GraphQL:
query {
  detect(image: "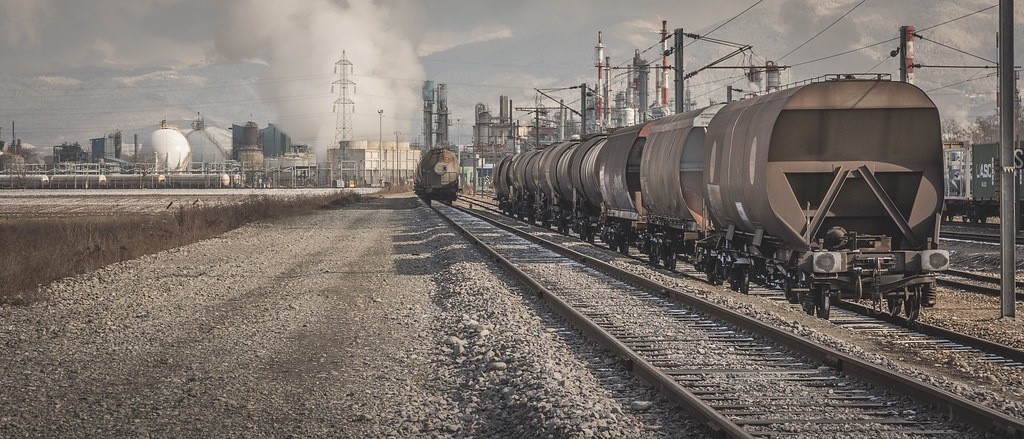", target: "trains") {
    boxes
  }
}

[414,147,462,205]
[492,72,948,321]
